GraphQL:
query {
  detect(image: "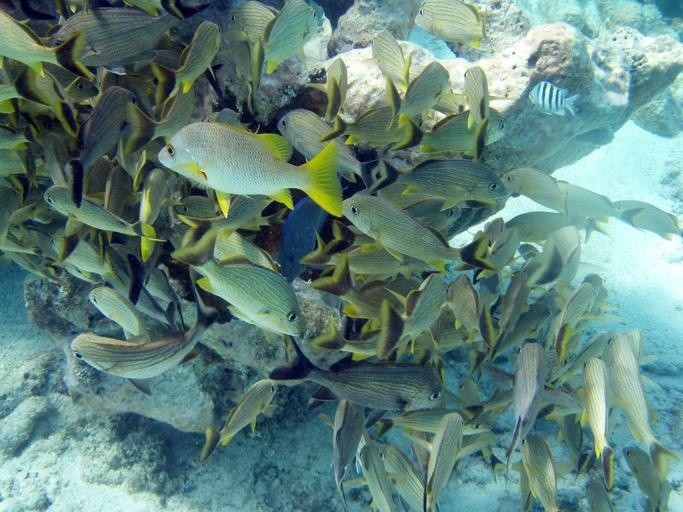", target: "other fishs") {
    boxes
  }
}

[0,0,682,512]
